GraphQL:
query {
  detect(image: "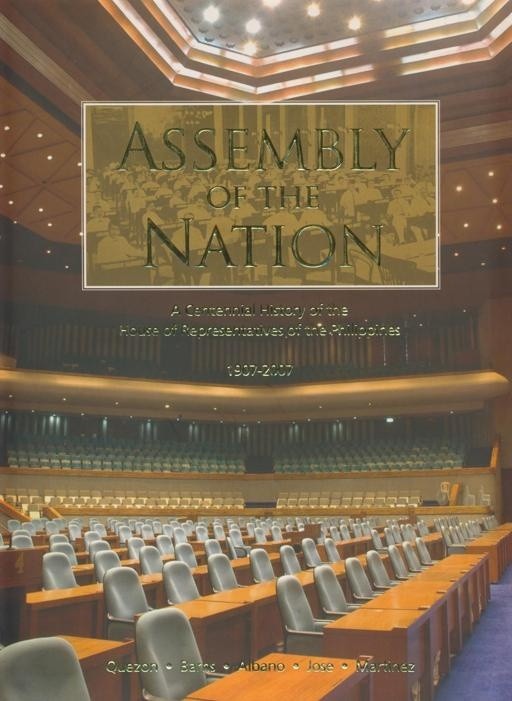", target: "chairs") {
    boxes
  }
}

[0,429,510,699]
